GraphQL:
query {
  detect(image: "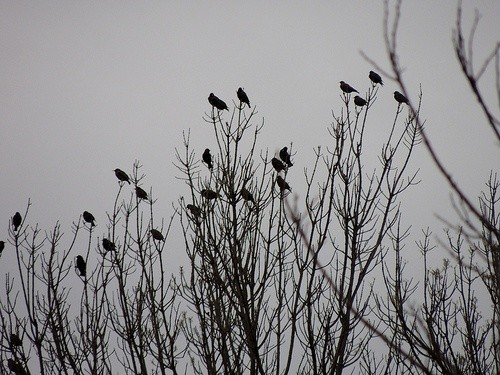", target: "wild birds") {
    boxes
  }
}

[201,147,214,170]
[6,357,26,375]
[9,333,23,347]
[100,238,118,252]
[0,240,7,254]
[275,175,292,193]
[112,168,132,186]
[82,210,96,227]
[391,90,410,106]
[236,87,251,109]
[207,92,231,112]
[338,80,360,95]
[353,95,369,107]
[240,186,257,205]
[11,211,23,233]
[148,228,165,242]
[133,186,149,200]
[278,146,294,168]
[200,188,223,201]
[271,157,287,173]
[368,70,385,87]
[186,203,203,217]
[75,254,87,277]
